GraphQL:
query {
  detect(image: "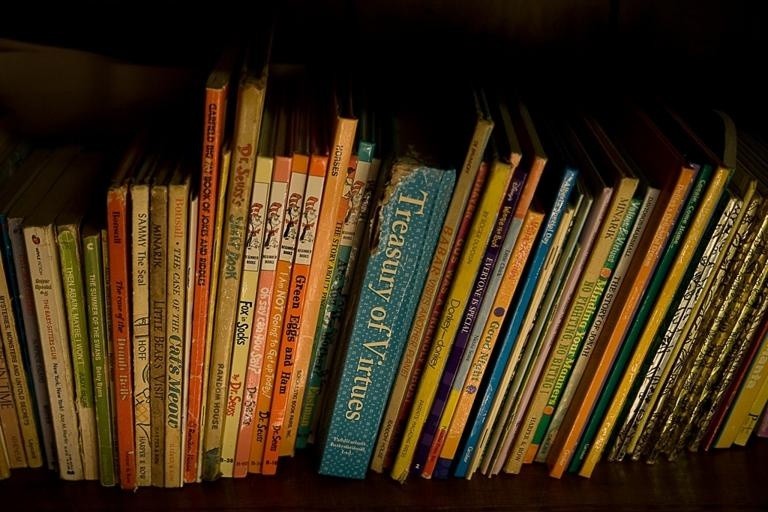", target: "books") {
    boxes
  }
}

[1,30,766,492]
